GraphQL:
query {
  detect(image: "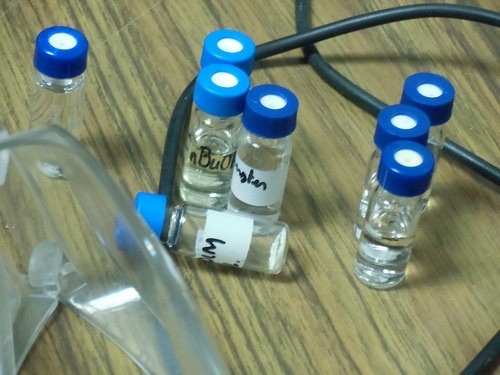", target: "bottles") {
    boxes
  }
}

[115,192,292,272]
[173,31,299,230]
[29,27,91,180]
[354,68,456,289]
